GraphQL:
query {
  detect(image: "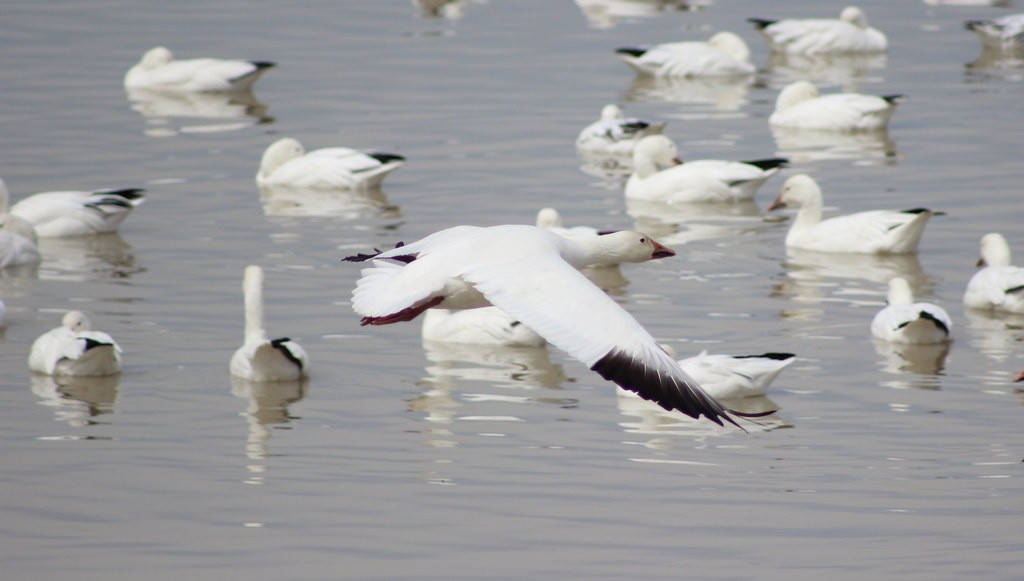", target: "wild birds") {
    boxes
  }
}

[0,175,149,378]
[227,265,312,381]
[122,45,279,86]
[339,0,1024,435]
[253,137,406,185]
